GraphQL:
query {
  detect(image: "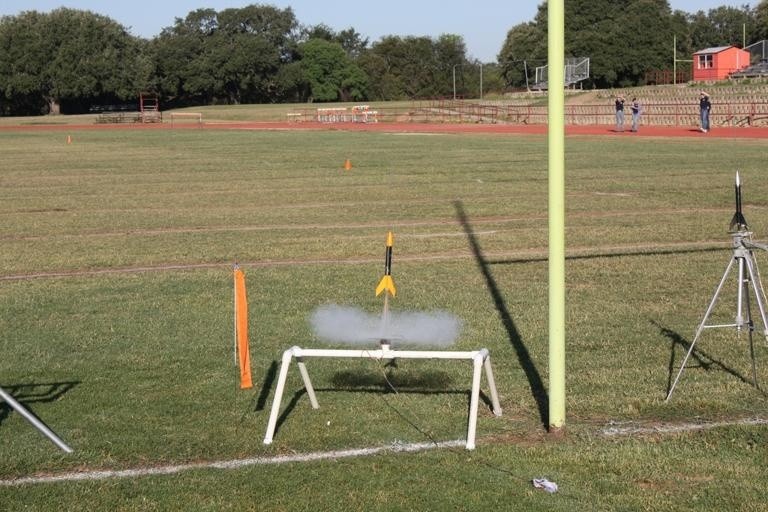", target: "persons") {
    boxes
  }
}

[695,91,711,133]
[614,93,625,130]
[628,96,641,131]
